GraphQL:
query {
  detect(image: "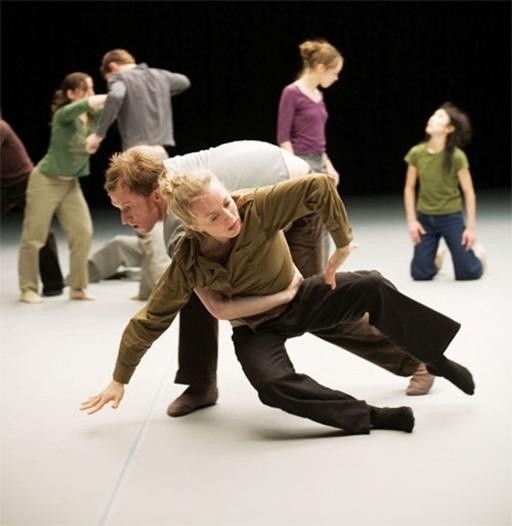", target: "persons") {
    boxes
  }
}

[275,36,345,272]
[83,48,191,301]
[61,234,143,286]
[104,139,331,419]
[77,172,476,436]
[17,72,108,304]
[0,116,65,297]
[401,102,488,281]
